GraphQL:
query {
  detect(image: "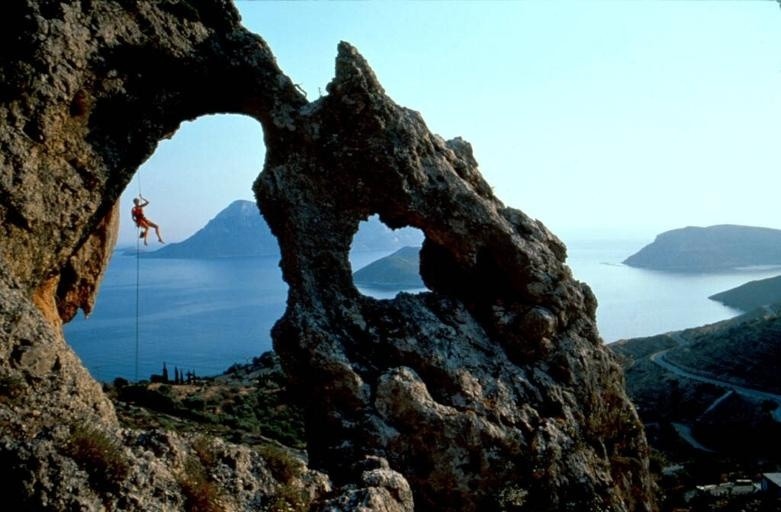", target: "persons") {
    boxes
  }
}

[132,193,165,247]
